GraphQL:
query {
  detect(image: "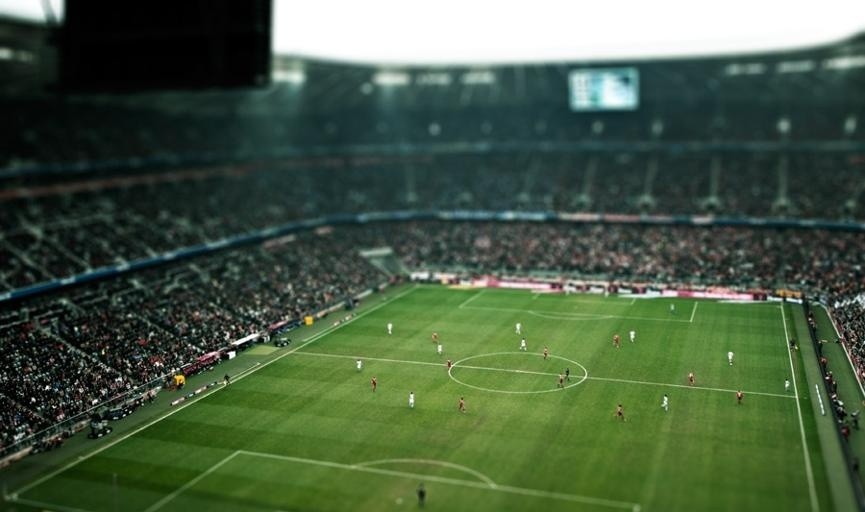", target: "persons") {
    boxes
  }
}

[783,379,790,391]
[805,297,865,476]
[613,403,626,421]
[789,337,796,352]
[436,342,442,356]
[405,100,865,295]
[515,323,521,336]
[542,346,549,360]
[611,334,621,351]
[555,374,564,389]
[628,329,635,343]
[520,338,527,351]
[661,393,669,412]
[737,390,744,405]
[431,330,437,343]
[459,397,465,413]
[688,372,694,385]
[408,391,415,408]
[563,367,570,382]
[447,358,452,370]
[1,109,406,467]
[727,351,734,366]
[415,483,427,509]
